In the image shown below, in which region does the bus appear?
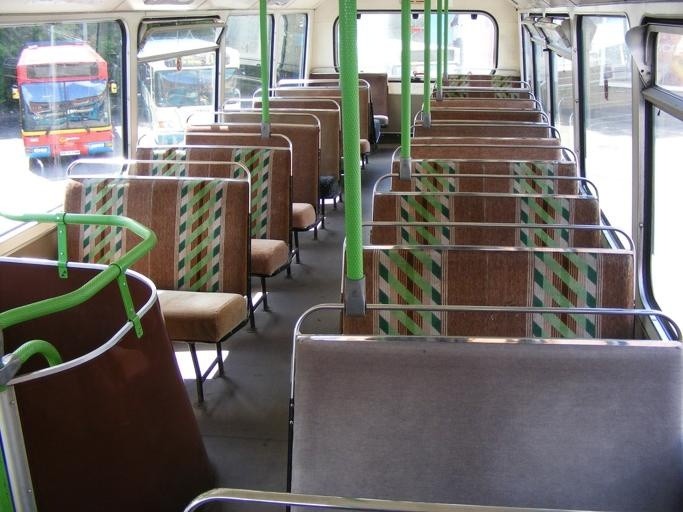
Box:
[142,40,241,144]
[10,37,118,175]
